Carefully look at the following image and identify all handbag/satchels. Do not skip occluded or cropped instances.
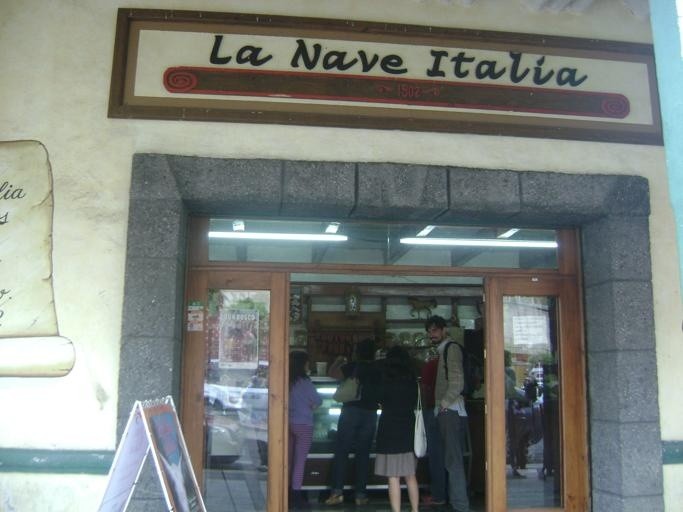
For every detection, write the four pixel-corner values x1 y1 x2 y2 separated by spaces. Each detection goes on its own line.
335 367 362 403
443 341 483 398
411 405 428 460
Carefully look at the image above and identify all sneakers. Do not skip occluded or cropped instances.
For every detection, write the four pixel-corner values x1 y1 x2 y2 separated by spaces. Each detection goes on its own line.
323 494 371 506
423 496 445 507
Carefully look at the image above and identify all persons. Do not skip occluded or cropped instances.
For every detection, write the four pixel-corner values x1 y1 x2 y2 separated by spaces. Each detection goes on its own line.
158 449 190 512
289 350 322 491
374 346 420 512
204 362 268 473
503 350 552 480
419 315 484 512
326 338 383 506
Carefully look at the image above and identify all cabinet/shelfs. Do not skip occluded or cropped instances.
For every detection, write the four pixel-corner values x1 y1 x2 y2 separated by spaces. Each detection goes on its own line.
289 294 310 352
295 384 431 490
380 297 459 362
